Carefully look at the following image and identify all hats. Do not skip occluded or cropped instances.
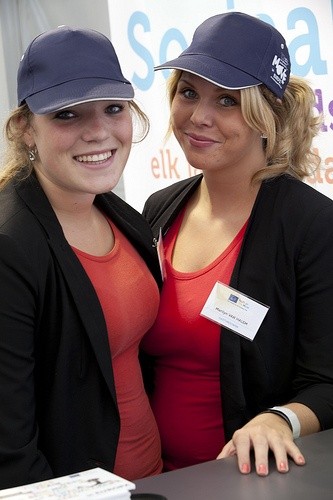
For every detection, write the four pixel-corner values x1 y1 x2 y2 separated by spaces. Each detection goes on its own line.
152 11 291 99
16 24 134 115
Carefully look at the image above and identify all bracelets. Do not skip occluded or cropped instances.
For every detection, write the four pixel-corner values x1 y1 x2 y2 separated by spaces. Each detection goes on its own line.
269 406 301 440
259 409 293 432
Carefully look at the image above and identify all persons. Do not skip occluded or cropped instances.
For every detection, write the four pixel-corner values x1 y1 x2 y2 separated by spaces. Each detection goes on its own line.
0 25 162 481
141 12 333 476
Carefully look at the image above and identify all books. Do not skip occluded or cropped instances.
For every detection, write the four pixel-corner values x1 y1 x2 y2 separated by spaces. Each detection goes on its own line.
0 467 137 500
